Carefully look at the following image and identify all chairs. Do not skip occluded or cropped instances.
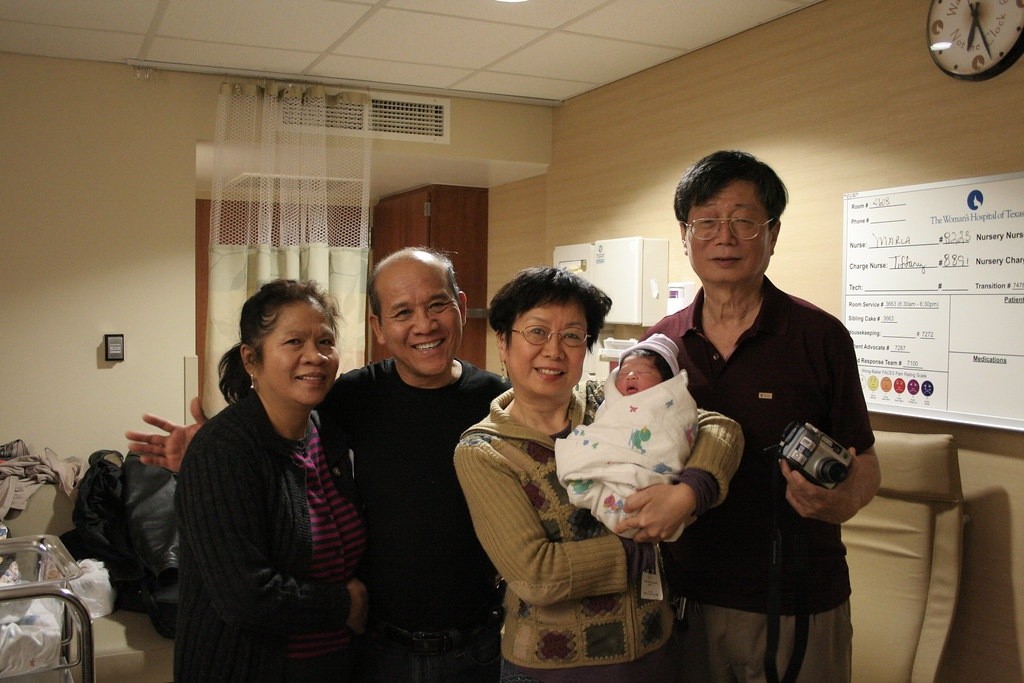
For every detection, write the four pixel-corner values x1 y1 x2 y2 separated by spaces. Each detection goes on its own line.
842 434 968 681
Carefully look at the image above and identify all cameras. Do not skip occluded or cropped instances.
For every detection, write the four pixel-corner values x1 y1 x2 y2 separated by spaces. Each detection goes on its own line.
777 420 854 490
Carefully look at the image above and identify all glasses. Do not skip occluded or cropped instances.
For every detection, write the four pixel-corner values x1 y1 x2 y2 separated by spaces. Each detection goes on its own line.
506 325 591 347
680 216 774 241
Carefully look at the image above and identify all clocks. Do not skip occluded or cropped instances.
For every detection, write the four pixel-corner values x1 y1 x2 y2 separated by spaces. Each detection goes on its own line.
928 0 1024 81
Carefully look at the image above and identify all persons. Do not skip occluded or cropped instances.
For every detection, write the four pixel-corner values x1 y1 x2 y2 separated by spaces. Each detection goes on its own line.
555 332 698 543
454 266 746 683
638 151 882 683
174 278 369 683
125 247 513 683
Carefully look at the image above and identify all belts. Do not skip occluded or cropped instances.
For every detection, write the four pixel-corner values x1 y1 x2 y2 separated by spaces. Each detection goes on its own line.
383 622 470 655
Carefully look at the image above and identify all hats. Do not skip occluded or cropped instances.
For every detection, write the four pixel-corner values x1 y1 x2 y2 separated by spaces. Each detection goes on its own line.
618 332 680 376
